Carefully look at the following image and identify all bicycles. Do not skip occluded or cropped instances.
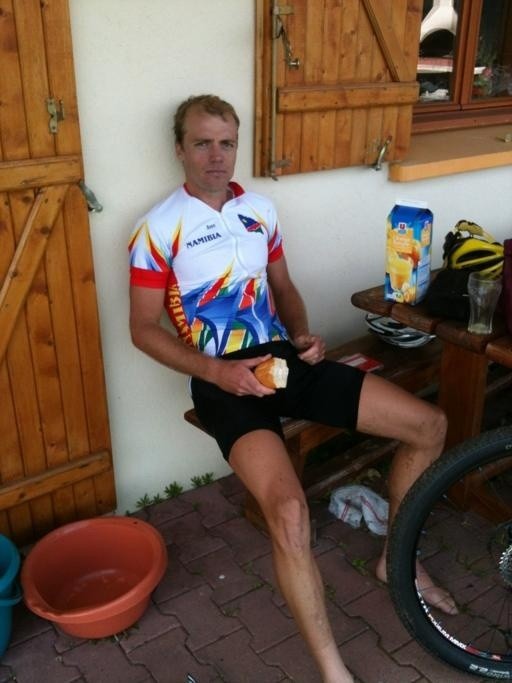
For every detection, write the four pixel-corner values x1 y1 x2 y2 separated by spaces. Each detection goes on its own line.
385 421 512 683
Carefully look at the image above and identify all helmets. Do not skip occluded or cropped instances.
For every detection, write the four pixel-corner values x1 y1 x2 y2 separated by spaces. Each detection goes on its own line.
440 220 504 289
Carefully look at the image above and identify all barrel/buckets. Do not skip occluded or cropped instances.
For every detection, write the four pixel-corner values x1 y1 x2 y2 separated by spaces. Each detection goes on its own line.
0 533 24 661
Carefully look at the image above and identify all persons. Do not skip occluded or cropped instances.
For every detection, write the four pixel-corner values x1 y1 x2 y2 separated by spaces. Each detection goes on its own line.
127 94 459 683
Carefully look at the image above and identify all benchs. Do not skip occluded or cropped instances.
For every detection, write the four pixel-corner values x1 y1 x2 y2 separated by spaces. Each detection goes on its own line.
182 336 445 549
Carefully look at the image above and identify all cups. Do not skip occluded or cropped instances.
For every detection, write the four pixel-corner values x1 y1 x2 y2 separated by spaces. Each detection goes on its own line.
466 269 503 335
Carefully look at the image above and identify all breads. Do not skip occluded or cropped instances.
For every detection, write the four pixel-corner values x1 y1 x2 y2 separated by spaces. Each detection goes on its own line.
254 357 289 389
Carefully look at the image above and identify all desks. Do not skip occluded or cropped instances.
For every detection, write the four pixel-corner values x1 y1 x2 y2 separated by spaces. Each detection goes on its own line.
350 268 512 526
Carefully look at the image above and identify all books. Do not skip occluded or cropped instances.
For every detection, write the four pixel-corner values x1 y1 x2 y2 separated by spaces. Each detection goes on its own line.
335 352 384 373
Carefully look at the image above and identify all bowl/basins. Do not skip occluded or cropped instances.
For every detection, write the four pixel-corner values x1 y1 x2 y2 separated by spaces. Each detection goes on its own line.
19 515 170 638
1 532 24 659
